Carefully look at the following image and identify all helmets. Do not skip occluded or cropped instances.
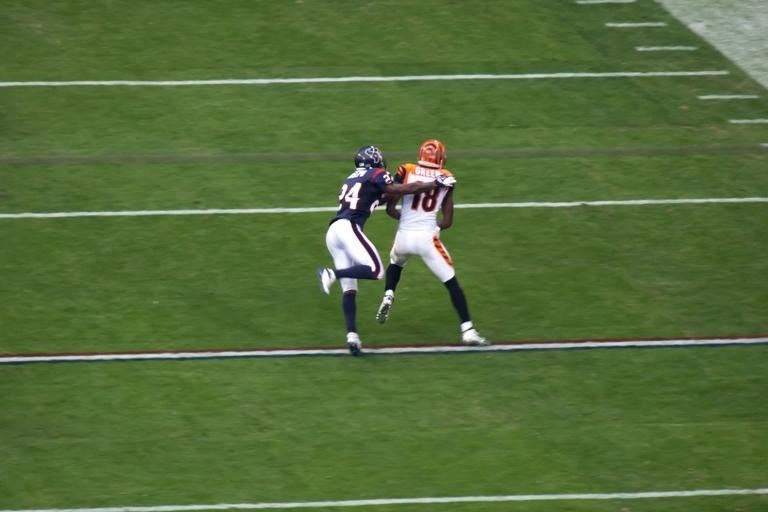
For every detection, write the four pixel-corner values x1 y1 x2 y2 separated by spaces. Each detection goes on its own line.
417 138 447 168
354 145 386 170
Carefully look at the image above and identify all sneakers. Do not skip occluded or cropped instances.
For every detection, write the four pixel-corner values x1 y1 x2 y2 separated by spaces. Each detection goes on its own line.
318 266 337 297
375 299 393 324
461 333 491 346
347 338 362 357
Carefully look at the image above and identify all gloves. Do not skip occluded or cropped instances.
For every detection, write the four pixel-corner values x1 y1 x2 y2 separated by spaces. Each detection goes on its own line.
436 174 457 188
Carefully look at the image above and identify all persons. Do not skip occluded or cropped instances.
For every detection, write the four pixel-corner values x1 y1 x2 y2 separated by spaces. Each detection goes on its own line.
375 139 490 347
319 144 459 359
